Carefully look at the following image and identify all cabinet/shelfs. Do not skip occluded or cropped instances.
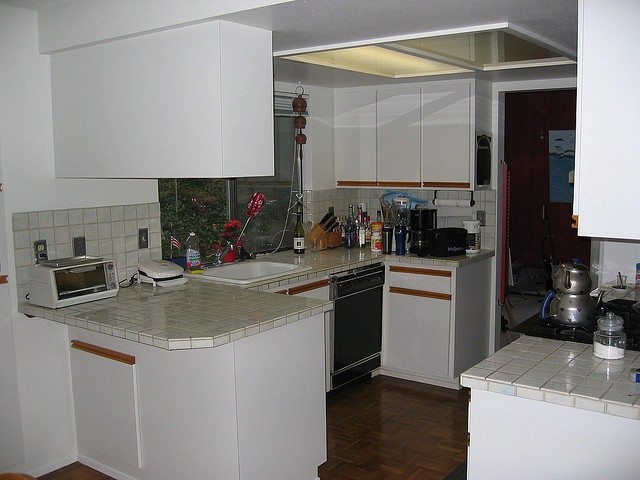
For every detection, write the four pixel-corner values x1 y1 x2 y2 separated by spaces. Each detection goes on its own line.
386 266 452 378
67 341 143 469
422 88 472 191
377 90 421 188
288 280 330 302
332 90 377 188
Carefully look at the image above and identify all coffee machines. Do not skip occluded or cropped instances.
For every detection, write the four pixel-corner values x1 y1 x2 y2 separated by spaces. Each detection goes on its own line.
408 208 437 257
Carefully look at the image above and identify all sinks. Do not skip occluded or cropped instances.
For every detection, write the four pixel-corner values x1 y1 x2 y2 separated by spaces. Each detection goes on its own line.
202 261 313 282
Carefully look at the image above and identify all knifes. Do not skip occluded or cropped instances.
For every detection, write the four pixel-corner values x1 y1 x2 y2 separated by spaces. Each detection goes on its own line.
320 212 339 234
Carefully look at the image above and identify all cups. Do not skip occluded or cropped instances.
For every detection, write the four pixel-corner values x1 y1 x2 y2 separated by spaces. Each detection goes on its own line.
617 275 627 289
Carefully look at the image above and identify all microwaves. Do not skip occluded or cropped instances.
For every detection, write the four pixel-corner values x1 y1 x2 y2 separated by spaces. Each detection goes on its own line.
28 254 121 310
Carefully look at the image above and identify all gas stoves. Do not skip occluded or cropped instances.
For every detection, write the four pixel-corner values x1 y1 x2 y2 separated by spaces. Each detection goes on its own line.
505 296 640 352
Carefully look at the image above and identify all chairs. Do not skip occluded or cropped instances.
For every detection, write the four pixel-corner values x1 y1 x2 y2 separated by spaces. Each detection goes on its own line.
506 259 541 299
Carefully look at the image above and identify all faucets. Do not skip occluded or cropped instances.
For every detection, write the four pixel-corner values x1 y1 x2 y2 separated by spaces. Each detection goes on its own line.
215 240 233 263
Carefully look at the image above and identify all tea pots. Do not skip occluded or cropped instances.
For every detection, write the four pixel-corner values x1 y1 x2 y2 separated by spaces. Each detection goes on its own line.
549 258 592 295
539 290 606 326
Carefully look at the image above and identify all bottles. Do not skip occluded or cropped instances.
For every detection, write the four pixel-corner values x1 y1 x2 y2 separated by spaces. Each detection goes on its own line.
377 210 382 222
357 205 364 223
365 215 372 244
348 204 355 224
382 226 393 254
293 202 305 254
395 225 407 256
345 219 354 248
358 222 367 248
370 232 383 253
462 220 482 254
593 311 628 359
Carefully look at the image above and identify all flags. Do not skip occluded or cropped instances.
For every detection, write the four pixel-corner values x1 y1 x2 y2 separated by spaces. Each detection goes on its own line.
171 226 182 253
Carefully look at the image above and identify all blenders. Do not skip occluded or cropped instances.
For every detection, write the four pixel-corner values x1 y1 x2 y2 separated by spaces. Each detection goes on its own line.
388 197 414 253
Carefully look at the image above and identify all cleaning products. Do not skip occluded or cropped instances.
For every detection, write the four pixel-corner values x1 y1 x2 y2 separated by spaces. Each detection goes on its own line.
186 232 202 271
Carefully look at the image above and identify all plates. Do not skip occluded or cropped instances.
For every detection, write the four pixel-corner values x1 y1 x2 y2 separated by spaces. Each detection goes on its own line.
610 285 632 293
